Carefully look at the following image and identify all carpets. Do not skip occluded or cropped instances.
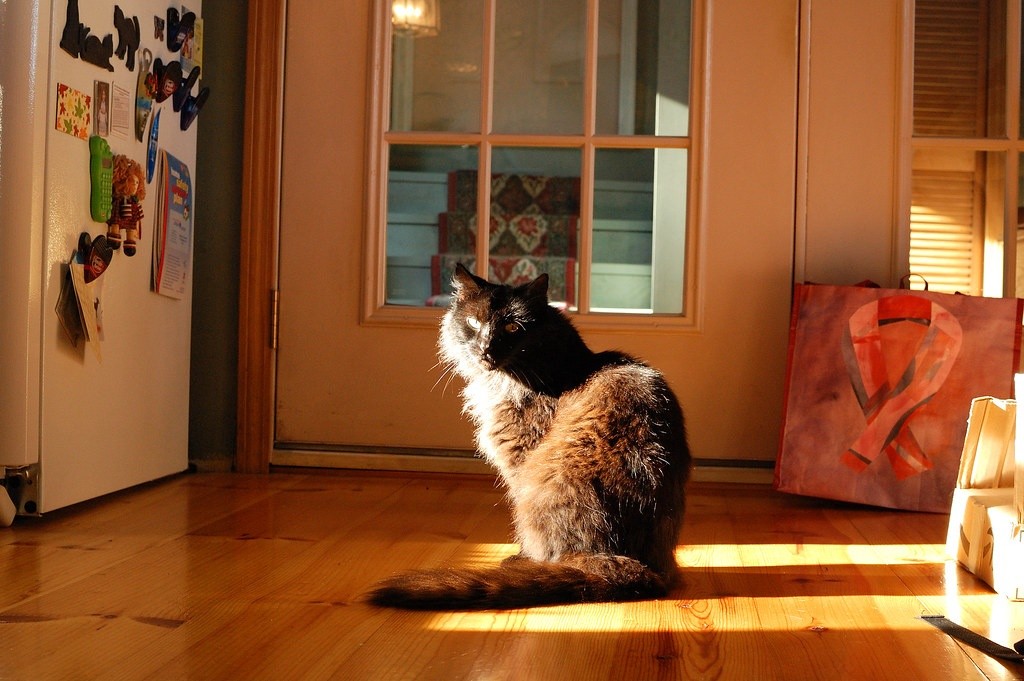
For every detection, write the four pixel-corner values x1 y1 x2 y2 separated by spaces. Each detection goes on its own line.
431 170 581 308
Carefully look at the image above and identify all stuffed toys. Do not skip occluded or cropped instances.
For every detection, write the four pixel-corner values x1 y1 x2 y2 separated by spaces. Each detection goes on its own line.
108 154 146 258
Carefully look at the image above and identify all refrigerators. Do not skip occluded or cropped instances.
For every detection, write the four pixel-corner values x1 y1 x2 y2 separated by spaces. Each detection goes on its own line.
0 1 205 521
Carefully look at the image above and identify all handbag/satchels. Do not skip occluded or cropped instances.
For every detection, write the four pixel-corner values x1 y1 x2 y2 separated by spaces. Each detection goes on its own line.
775 274 1024 512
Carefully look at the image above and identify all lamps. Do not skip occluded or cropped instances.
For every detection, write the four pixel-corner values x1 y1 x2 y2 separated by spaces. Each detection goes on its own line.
390 0 441 40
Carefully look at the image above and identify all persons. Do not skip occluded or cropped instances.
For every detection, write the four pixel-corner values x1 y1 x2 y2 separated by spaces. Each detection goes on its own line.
182 28 194 59
98 86 108 135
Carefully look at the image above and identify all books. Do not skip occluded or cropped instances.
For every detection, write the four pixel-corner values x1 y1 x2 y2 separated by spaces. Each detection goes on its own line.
153 149 192 299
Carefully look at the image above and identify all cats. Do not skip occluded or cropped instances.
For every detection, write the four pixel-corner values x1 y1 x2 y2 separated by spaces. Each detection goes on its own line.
355 263 691 612
